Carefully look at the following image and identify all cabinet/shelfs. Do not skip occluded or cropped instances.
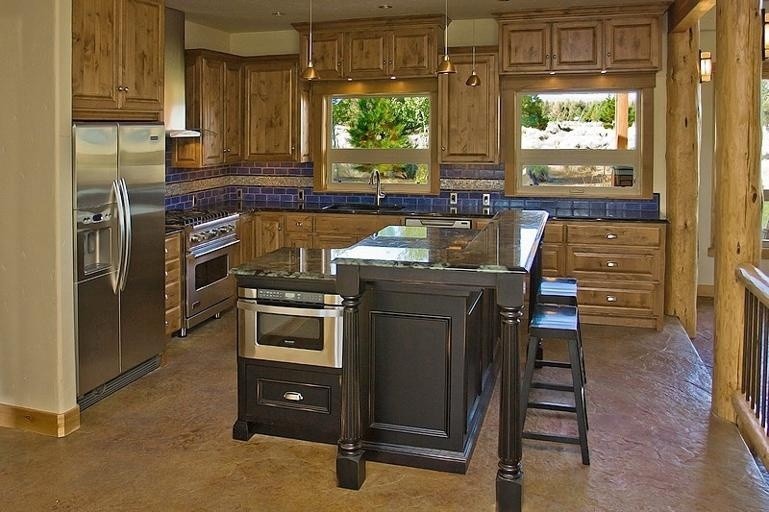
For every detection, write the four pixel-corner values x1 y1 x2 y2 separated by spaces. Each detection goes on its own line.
603 15 663 73
498 18 603 73
245 57 310 164
255 209 284 257
559 221 669 331
164 234 184 334
436 53 498 164
315 212 404 250
285 211 313 250
171 48 243 169
344 24 444 81
240 210 256 264
72 0 166 123
540 223 568 277
300 32 344 80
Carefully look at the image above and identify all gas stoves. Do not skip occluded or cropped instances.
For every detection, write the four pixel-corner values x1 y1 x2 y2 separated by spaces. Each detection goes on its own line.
165 207 234 227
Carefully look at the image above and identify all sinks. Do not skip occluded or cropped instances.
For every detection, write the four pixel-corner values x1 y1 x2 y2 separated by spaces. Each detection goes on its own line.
379 206 404 213
322 204 380 212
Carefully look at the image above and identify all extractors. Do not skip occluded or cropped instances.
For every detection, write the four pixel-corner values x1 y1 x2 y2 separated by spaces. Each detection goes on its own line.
165 20 202 139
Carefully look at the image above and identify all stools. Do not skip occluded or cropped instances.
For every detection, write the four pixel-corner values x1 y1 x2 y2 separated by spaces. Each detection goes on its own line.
521 305 590 464
536 277 587 385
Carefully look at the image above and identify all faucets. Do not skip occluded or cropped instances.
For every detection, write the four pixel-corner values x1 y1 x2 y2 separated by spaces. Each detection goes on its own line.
370 169 385 206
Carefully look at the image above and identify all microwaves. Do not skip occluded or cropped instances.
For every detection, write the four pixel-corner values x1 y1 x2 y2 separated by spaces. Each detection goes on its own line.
236 290 344 373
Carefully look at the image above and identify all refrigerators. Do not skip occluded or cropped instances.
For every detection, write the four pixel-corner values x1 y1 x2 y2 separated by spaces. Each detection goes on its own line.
71 120 166 413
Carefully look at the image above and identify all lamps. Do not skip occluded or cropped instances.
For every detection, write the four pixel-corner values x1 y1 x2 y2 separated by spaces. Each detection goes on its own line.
464 18 481 86
700 51 712 81
301 0 321 80
435 1 458 75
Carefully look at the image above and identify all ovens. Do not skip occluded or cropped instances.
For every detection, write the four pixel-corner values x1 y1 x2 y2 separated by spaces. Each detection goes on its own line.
183 217 240 330
